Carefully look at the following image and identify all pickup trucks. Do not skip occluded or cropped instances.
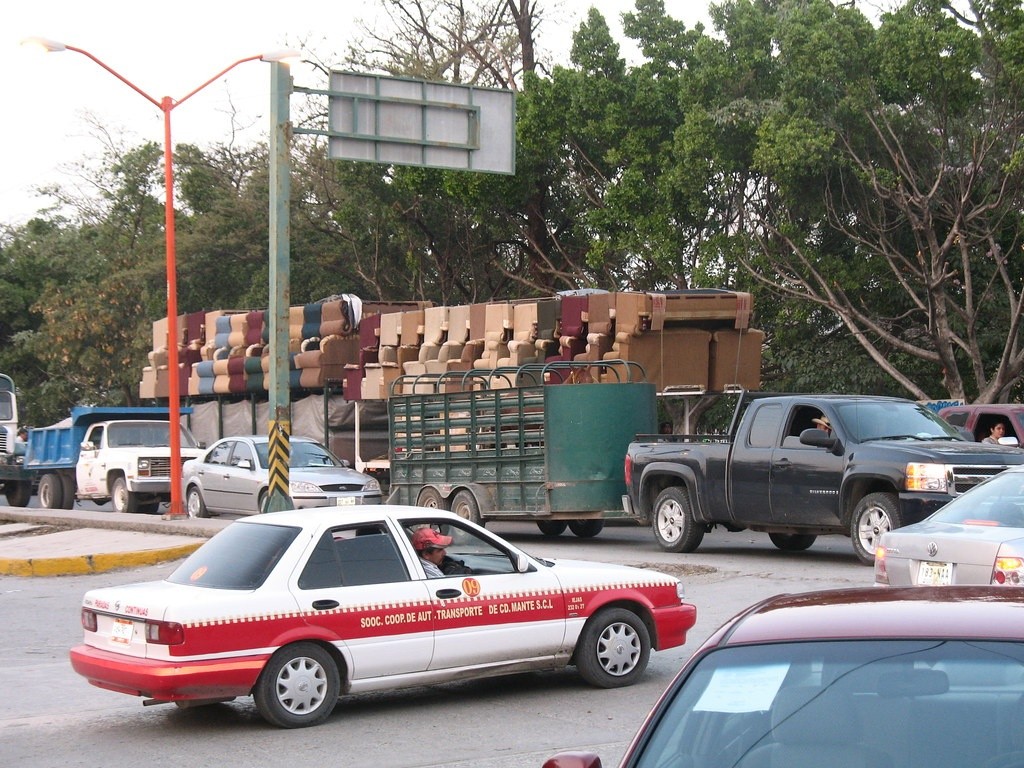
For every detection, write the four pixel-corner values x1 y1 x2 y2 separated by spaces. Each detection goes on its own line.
621 392 1024 568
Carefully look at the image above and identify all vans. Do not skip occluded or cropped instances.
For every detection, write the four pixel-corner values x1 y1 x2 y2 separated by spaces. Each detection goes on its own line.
935 403 1024 452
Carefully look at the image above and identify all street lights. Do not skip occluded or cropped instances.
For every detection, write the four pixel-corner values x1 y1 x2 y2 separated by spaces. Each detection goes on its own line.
17 36 308 519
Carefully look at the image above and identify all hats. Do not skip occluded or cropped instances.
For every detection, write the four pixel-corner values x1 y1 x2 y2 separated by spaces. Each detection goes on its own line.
412 528 452 549
812 415 832 430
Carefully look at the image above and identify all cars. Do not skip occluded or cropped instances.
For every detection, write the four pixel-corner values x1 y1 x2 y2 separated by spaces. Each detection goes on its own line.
69 504 700 729
542 586 1024 768
180 435 382 517
870 460 1024 590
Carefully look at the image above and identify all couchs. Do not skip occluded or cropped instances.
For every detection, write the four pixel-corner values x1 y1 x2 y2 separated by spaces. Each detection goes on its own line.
139 287 764 403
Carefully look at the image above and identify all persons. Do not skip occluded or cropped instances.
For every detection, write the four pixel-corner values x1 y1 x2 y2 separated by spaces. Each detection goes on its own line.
982 419 1006 445
817 416 832 437
356 526 452 579
659 422 673 434
17 428 28 442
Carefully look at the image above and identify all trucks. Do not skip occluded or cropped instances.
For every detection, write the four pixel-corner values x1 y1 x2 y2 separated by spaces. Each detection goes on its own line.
21 406 213 514
0 373 34 509
